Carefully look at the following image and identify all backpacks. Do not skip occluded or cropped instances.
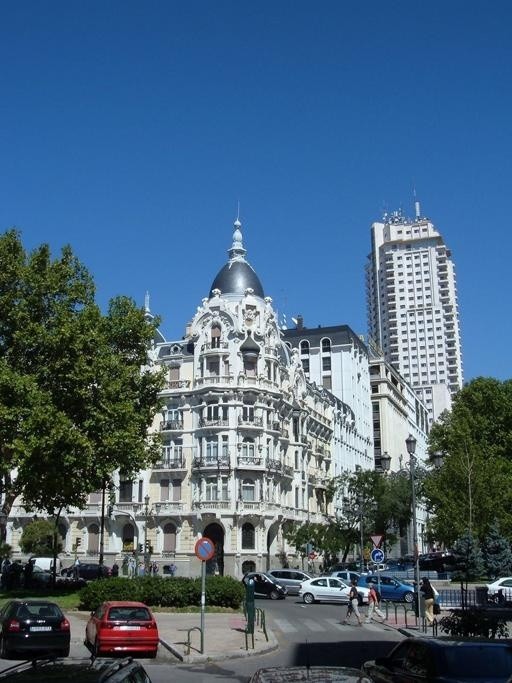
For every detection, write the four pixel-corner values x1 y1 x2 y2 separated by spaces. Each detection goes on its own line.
373 588 381 603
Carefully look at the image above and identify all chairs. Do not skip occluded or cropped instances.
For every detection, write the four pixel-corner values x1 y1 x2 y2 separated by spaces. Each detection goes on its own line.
131 611 146 618
38 608 51 617
110 611 120 618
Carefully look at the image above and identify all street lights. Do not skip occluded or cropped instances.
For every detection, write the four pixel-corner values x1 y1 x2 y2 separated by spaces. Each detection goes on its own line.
380 434 444 631
129 495 161 576
342 492 381 573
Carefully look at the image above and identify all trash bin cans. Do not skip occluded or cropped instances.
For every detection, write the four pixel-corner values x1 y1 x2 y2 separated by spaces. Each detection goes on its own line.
475 586 488 605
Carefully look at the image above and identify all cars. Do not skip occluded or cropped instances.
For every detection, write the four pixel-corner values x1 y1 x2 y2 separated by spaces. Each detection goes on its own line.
62 562 111 580
486 576 512 604
242 563 425 604
0 598 158 683
20 565 53 584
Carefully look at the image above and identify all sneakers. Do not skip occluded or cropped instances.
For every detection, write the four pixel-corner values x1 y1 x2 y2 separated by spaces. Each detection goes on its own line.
342 616 387 625
421 620 437 627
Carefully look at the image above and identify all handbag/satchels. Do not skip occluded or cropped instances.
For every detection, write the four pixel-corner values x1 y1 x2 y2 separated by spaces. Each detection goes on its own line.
434 603 441 614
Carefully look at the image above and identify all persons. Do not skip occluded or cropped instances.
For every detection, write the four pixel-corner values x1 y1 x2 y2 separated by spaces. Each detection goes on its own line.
365 581 385 624
340 579 363 625
24 557 36 588
72 554 82 578
419 576 436 627
111 558 179 579
